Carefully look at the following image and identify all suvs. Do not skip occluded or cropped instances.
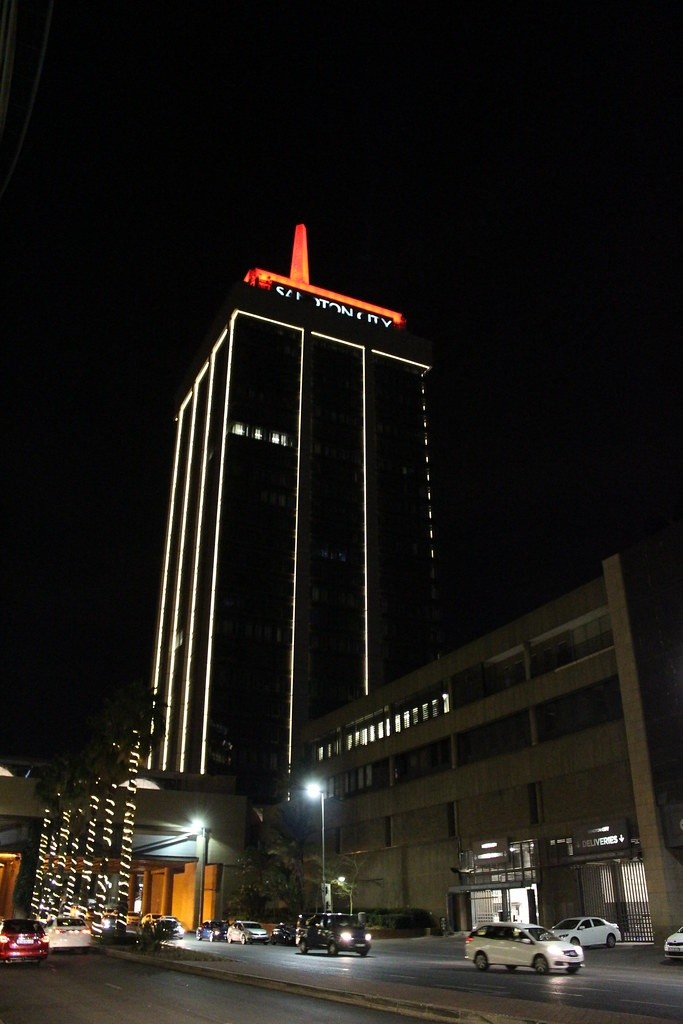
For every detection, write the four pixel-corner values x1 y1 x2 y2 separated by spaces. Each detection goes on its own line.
295 912 372 957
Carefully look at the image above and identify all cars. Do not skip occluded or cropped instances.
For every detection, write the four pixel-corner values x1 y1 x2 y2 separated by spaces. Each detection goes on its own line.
0 918 51 966
465 922 586 976
270 924 297 947
197 920 230 943
539 917 622 951
40 916 92 955
226 921 269 945
664 928 683 960
142 913 185 940
102 908 140 926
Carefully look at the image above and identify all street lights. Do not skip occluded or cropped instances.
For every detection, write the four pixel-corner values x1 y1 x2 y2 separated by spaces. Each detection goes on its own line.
306 782 328 915
190 817 208 928
